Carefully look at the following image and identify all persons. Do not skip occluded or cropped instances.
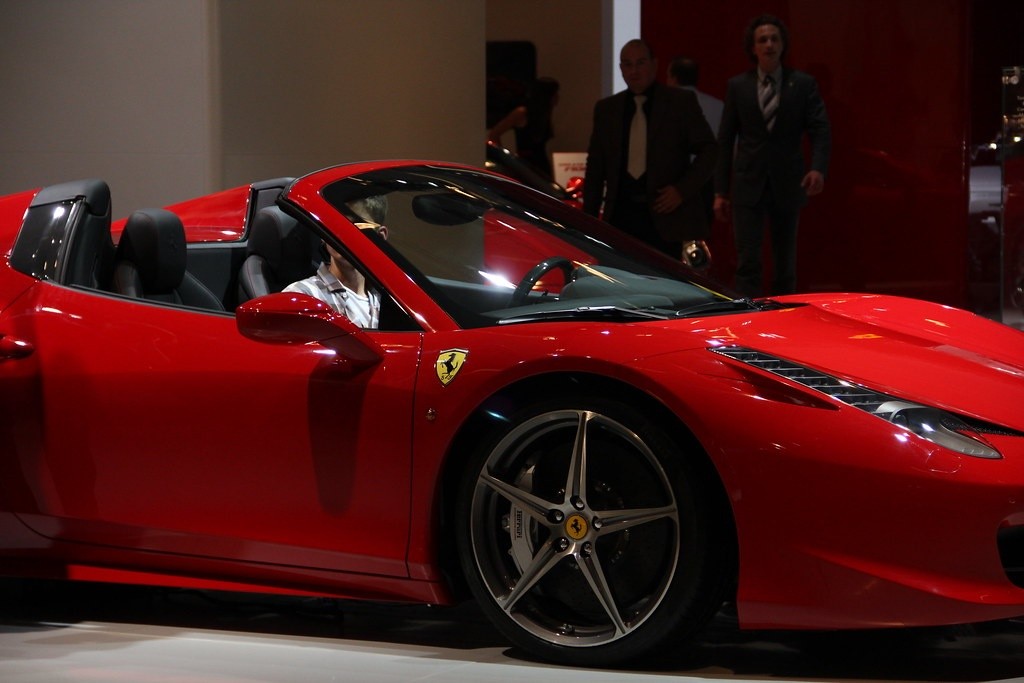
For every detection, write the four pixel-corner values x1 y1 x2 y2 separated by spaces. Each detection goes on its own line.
663 57 726 263
581 38 717 263
280 194 389 328
487 76 560 185
711 16 833 299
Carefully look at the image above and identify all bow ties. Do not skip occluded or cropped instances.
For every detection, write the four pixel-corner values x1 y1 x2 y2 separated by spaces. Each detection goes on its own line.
626 95 647 180
762 74 779 133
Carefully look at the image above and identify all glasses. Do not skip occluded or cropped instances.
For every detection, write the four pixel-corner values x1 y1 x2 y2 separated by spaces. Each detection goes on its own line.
619 57 649 70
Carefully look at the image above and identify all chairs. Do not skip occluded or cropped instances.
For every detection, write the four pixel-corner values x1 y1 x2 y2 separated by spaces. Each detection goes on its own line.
235 205 325 308
108 207 226 317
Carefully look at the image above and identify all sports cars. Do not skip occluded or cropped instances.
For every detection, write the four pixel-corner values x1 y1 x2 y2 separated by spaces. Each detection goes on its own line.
2 158 1024 663
